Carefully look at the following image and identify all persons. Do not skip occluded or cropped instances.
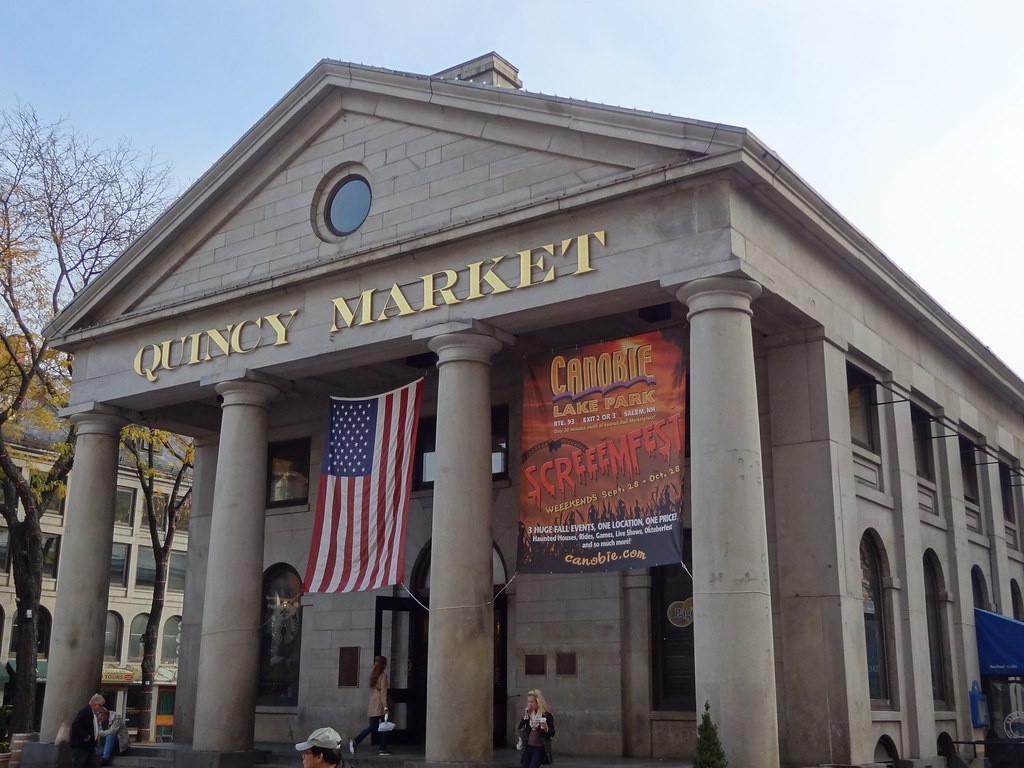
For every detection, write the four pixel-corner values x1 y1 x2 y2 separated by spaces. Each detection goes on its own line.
349 656 392 756
69 693 105 768
294 726 355 768
517 688 556 768
94 707 130 768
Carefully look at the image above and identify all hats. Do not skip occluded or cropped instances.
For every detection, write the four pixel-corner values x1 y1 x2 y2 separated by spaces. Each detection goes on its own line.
295 727 342 751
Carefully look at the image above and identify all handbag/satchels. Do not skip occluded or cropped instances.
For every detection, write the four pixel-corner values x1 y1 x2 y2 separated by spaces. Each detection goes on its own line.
515 737 523 751
378 718 396 731
54 720 72 745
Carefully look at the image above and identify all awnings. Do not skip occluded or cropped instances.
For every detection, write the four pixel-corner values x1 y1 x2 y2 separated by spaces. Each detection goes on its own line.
974 606 1024 678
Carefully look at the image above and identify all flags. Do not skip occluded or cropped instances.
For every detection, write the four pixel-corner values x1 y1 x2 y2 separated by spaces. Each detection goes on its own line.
300 377 423 593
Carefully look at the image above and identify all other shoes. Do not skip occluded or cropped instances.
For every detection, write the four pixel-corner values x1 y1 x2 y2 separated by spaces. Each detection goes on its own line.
378 750 392 756
348 740 356 755
